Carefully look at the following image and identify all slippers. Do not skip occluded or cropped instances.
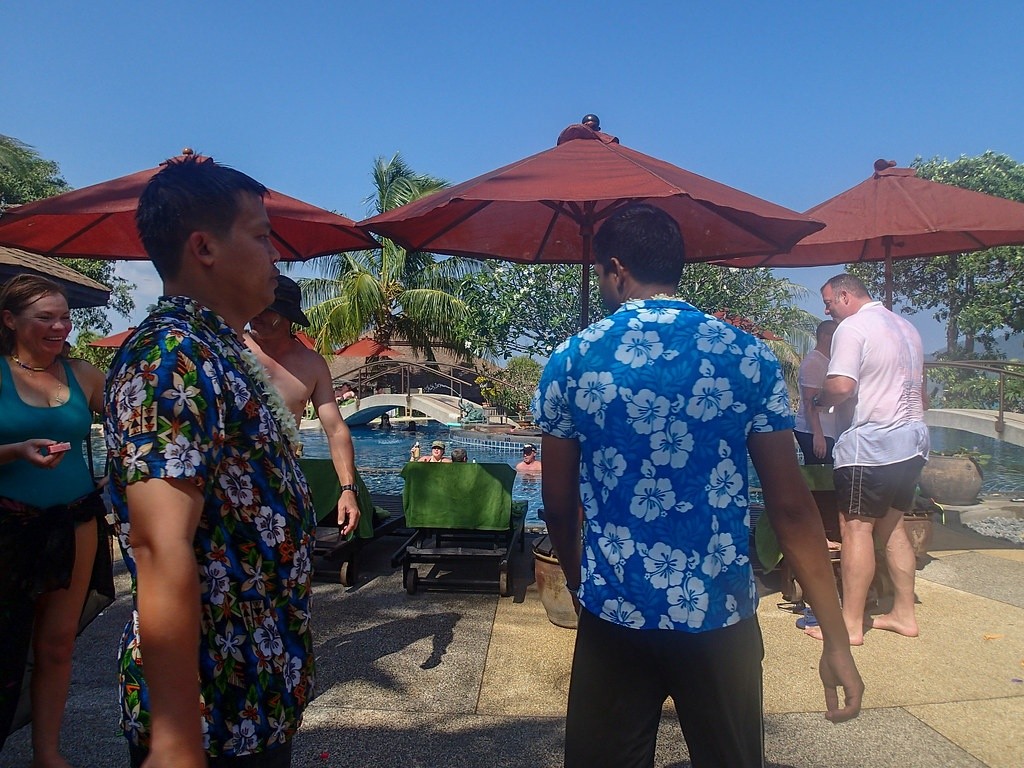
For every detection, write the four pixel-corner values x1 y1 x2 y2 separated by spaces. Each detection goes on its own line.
794 605 820 629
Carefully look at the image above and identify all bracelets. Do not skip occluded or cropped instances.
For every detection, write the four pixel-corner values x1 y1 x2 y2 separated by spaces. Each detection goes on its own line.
341 482 359 497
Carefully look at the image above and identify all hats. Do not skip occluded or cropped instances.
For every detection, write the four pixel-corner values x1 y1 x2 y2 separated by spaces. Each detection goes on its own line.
520 444 537 452
262 275 309 326
430 441 445 449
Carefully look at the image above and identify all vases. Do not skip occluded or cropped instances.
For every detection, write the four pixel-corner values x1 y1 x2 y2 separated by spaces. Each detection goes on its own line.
900 515 932 570
533 549 579 629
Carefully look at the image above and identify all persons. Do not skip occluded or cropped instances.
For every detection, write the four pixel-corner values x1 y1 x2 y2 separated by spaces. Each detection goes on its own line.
516 443 541 471
411 440 468 462
103 150 317 768
792 320 839 465
802 274 931 648
242 274 361 535
0 274 108 768
533 202 866 768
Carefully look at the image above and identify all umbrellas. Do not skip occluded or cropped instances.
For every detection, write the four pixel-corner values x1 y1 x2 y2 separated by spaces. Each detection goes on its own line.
712 310 784 339
708 158 1024 312
334 337 407 356
353 113 829 332
0 147 387 263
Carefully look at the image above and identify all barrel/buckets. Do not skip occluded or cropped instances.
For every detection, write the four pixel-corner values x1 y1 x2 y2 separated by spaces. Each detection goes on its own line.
532 547 579 630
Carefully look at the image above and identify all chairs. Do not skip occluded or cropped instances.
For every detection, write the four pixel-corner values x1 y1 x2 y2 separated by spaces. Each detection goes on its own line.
295 458 411 587
747 464 878 614
391 462 529 596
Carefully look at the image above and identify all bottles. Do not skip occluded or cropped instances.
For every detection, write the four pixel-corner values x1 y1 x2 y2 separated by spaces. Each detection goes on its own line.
413 441 420 458
473 457 476 463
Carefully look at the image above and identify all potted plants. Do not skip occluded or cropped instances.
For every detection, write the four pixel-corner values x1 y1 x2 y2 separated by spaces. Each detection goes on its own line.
918 444 992 506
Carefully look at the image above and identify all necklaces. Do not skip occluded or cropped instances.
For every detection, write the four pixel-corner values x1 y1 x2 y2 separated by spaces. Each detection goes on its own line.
19 363 66 403
11 352 58 371
